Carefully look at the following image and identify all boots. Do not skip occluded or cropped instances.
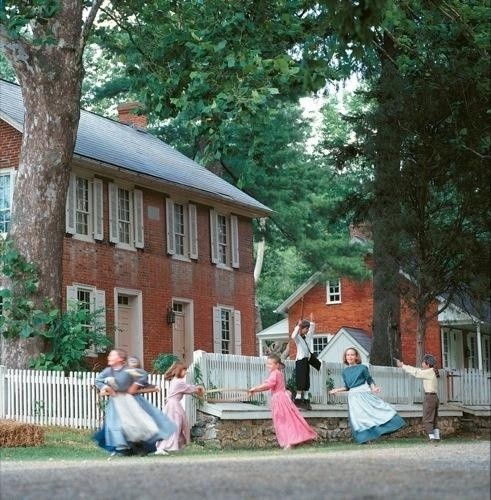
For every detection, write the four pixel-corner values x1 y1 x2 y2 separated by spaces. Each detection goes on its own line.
294 399 312 410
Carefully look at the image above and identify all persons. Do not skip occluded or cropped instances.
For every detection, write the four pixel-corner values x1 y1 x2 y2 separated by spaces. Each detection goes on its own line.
91 355 162 457
328 346 408 446
391 352 444 442
92 347 180 459
248 353 322 451
152 359 207 457
290 318 318 411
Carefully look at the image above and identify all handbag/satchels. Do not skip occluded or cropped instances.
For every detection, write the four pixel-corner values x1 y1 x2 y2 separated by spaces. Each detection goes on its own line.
308 353 321 370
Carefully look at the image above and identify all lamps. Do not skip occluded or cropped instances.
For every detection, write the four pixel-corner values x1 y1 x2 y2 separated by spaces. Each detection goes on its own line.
166 301 176 325
